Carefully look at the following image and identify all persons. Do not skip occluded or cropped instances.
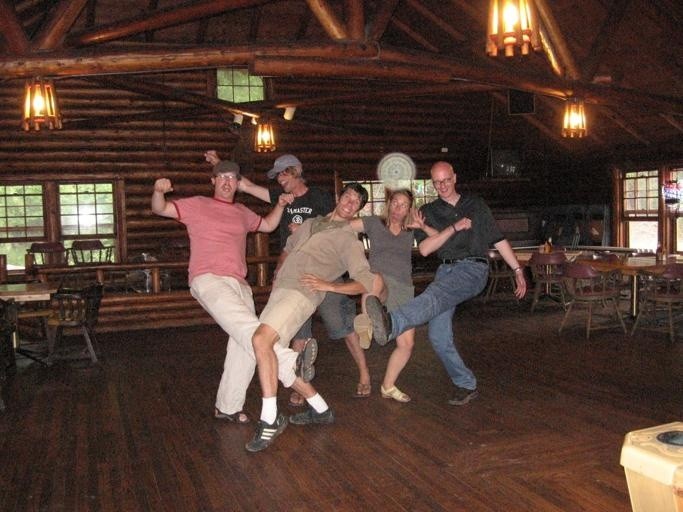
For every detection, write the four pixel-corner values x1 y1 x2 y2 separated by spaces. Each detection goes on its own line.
204 148 373 406
243 183 373 453
151 161 319 424
365 161 528 406
289 189 438 403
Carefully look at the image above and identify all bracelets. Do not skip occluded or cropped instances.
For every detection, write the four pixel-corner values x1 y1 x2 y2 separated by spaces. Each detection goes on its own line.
449 223 456 234
514 267 523 273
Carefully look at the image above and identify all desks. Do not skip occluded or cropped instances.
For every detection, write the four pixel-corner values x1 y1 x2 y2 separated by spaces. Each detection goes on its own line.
510 251 583 300
0 282 62 352
573 253 683 318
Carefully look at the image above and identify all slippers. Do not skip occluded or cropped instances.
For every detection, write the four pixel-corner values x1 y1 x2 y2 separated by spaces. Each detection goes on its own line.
287 390 308 409
350 382 372 399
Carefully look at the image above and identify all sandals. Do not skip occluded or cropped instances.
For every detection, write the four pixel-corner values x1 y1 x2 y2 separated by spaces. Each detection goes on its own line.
380 383 411 403
213 408 251 424
295 338 319 383
353 313 372 350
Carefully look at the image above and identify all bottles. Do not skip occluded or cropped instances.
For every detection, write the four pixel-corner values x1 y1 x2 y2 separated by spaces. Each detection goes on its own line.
548 238 552 253
544 235 550 253
656 241 662 261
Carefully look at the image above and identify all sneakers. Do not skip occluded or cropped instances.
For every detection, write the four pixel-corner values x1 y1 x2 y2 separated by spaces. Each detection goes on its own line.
448 387 480 406
244 411 290 453
289 406 336 425
365 294 392 348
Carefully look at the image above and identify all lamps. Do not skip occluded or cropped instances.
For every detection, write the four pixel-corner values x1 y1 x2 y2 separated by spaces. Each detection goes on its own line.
485 0 541 61
253 118 275 154
562 94 588 140
21 76 63 133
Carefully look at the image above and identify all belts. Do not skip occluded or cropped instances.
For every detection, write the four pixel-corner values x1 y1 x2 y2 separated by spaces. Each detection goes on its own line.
445 257 488 264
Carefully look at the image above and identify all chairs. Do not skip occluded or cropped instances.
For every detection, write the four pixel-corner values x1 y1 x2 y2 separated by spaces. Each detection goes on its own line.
537 245 566 254
604 254 629 314
630 263 683 342
482 250 524 307
558 263 627 340
635 252 656 257
27 242 69 283
71 241 116 264
530 251 570 316
47 282 105 363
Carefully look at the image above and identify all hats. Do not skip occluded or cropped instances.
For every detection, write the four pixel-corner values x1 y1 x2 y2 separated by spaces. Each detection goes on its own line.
267 153 302 180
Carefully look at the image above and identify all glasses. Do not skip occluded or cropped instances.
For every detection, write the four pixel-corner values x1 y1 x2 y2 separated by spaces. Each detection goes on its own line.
215 174 238 181
433 176 454 186
